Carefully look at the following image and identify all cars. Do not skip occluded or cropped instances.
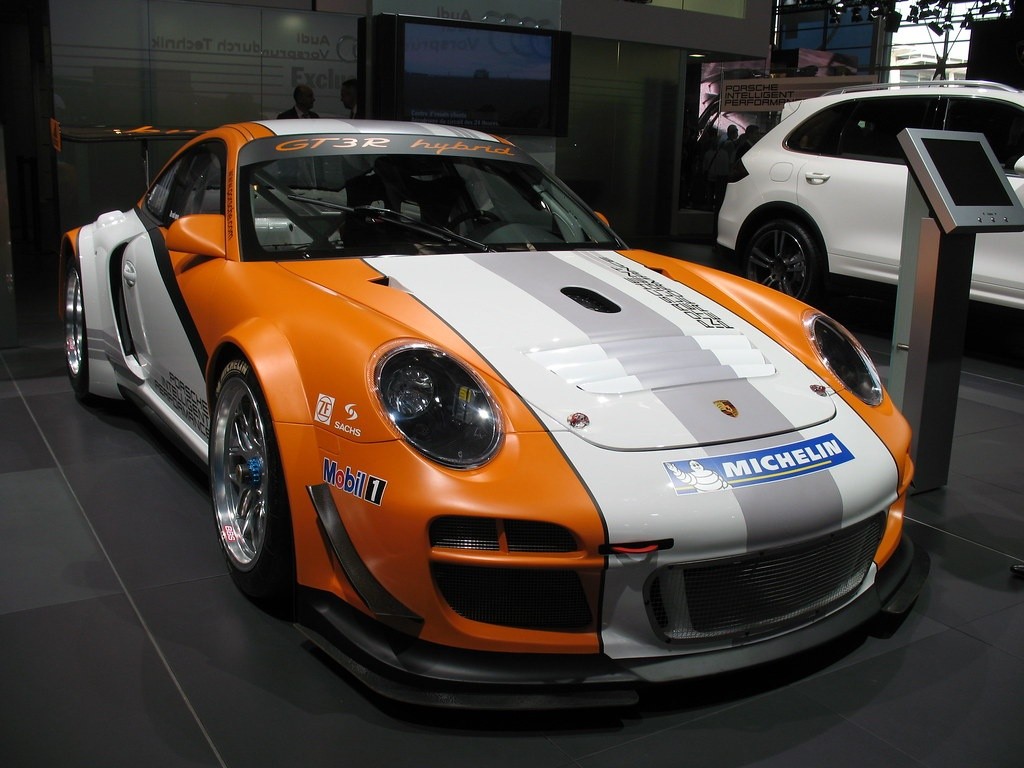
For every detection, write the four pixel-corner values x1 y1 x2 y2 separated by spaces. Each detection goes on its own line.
57 118 929 714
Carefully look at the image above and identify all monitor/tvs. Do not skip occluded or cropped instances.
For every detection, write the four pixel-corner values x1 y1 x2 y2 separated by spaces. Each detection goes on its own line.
896 128 1024 235
372 13 572 138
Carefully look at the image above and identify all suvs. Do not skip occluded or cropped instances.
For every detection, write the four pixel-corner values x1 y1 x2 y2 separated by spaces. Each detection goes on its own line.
711 79 1024 310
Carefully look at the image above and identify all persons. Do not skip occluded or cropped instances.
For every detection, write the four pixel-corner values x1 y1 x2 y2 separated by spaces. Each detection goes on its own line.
277 84 319 118
702 124 762 211
341 79 358 116
